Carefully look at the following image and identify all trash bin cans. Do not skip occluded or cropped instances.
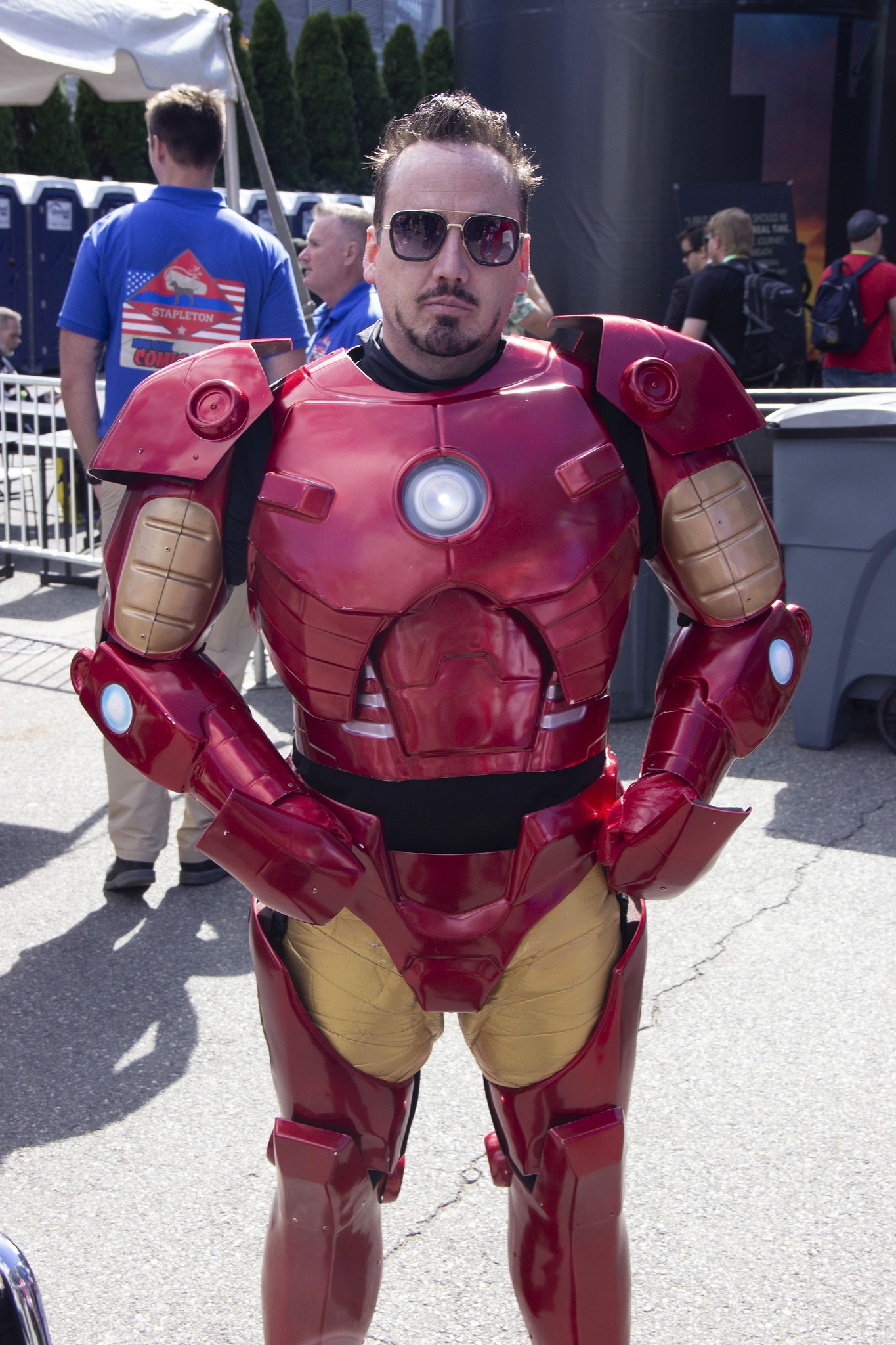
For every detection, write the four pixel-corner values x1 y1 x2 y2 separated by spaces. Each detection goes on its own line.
608 558 671 723
766 395 896 751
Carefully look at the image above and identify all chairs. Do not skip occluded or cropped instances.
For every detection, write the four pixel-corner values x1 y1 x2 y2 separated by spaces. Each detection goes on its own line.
0 468 38 546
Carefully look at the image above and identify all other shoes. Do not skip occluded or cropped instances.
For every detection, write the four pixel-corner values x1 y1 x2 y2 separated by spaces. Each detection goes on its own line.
104 857 157 889
179 858 230 885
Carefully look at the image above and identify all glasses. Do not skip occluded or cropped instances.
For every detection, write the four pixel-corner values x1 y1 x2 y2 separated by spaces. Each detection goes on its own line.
381 210 523 267
681 248 697 259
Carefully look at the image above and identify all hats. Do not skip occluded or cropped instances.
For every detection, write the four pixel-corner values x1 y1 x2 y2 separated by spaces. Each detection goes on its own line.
847 210 888 242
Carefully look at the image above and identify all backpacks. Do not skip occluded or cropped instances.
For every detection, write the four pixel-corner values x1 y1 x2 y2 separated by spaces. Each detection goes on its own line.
706 259 805 388
810 253 890 354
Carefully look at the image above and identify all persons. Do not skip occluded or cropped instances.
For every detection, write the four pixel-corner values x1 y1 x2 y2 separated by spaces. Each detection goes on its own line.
58 84 814 1345
0 307 101 523
660 209 896 387
